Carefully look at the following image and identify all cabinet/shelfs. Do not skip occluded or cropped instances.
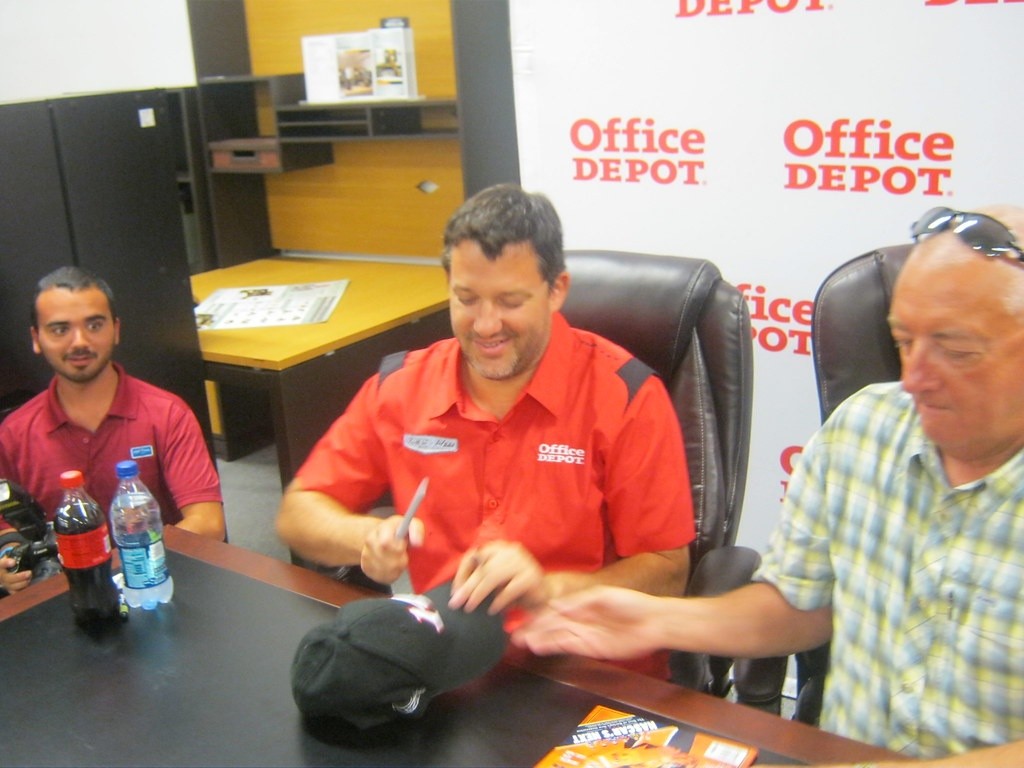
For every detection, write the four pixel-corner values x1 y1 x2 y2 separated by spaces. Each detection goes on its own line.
183 0 520 564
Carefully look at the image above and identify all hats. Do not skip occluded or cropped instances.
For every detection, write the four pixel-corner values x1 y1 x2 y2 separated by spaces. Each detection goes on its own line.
290 579 506 734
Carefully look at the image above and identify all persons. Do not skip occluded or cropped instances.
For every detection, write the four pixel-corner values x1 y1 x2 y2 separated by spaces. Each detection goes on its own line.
513 203 1024 768
275 183 698 682
0 265 226 596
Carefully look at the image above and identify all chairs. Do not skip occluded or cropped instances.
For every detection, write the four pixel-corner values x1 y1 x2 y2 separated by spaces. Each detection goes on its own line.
736 238 912 736
559 250 763 695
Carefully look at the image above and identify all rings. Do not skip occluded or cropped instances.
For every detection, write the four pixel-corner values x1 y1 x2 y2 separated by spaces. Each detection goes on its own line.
462 552 480 571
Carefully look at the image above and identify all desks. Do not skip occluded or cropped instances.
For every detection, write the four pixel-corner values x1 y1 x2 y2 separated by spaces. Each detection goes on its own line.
0 521 912 768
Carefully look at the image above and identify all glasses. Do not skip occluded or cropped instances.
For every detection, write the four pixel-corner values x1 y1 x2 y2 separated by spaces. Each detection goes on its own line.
911 206 1024 261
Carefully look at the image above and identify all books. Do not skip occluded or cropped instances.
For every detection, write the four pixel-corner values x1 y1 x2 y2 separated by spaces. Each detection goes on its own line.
532 705 758 768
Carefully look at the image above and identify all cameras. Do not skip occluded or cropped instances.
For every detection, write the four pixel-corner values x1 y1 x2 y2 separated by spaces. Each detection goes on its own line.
0 479 64 594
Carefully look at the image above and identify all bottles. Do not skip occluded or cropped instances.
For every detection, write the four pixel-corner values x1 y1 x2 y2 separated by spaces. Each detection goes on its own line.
109 459 173 610
53 470 120 639
